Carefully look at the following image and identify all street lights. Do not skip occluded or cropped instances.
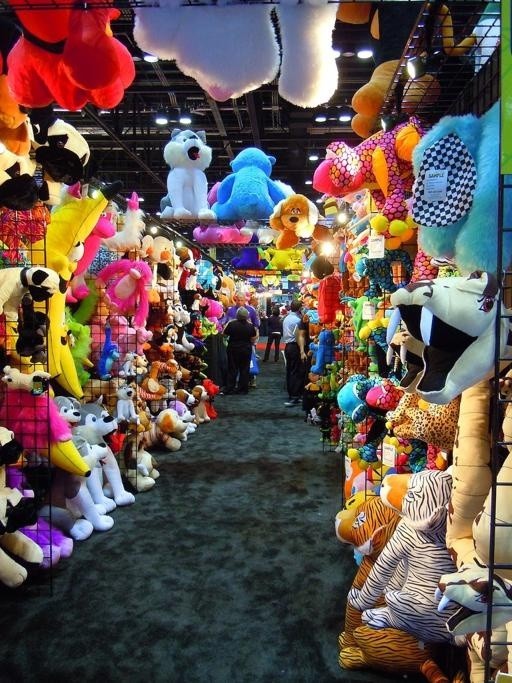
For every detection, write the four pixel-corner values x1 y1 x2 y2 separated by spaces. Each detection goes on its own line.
249 349 259 375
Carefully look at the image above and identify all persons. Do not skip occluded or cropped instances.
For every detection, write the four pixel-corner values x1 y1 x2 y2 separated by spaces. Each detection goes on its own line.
281 300 302 406
262 307 283 362
220 293 259 388
296 307 323 422
221 306 259 396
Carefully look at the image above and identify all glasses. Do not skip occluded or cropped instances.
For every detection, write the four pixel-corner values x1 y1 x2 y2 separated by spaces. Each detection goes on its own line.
379 113 411 130
407 52 444 78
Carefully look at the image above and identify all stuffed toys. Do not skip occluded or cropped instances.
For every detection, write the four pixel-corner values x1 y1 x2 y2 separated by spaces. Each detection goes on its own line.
212 147 287 223
2 74 258 587
131 3 340 108
271 56 509 683
6 1 135 114
336 2 479 59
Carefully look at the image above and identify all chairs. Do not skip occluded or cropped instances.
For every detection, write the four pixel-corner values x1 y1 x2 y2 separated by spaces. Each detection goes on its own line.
283 398 302 406
